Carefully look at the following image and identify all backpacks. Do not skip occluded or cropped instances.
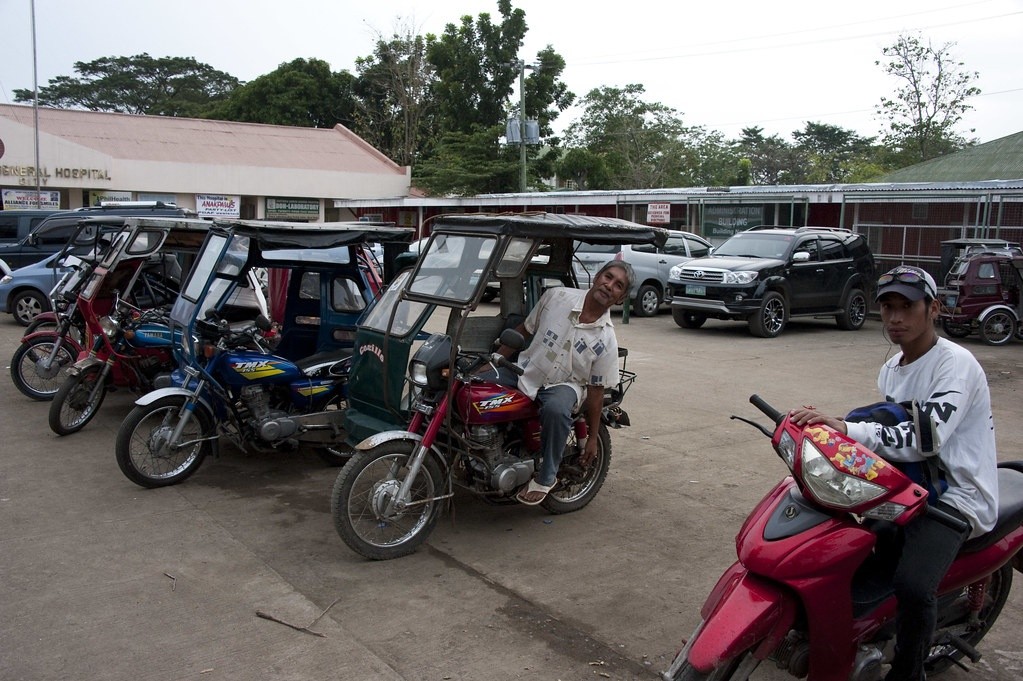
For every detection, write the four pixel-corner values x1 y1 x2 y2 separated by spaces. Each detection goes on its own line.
842 395 950 505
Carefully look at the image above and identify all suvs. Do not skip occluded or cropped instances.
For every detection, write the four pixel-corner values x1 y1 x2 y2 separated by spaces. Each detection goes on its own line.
665 224 879 338
0 201 198 272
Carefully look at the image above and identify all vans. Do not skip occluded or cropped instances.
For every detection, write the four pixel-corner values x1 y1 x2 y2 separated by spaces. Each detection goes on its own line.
0 211 68 243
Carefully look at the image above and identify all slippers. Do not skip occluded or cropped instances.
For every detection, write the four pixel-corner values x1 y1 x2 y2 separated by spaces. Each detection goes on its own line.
516 476 558 505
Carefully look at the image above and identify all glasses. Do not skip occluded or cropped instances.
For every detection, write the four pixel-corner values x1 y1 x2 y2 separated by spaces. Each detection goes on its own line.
877 273 937 299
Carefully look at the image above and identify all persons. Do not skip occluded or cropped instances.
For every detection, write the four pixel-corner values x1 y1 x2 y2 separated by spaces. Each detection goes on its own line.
790 265 999 681
475 260 636 505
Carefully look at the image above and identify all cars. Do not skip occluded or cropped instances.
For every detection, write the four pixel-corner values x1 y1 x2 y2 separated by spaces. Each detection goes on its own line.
0 247 89 328
572 232 716 316
939 252 1023 346
373 237 431 281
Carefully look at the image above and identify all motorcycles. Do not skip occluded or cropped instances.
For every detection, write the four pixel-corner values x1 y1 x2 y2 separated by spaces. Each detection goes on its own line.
10 213 673 561
658 394 1023 681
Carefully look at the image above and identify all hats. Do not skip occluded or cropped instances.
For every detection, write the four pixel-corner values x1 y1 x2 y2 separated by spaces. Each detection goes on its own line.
875 265 938 301
